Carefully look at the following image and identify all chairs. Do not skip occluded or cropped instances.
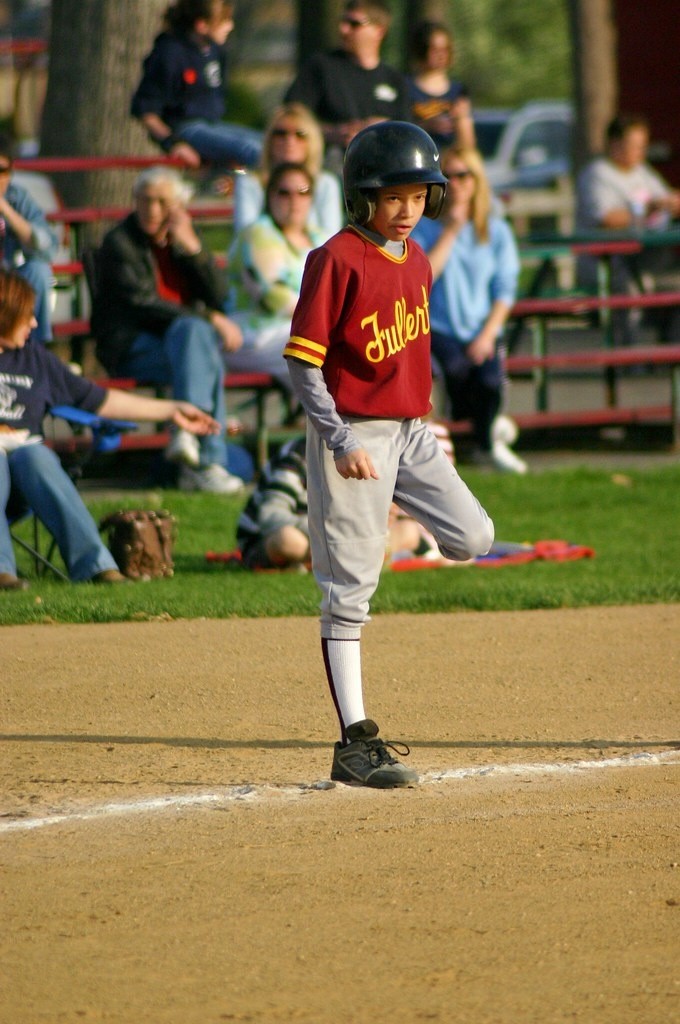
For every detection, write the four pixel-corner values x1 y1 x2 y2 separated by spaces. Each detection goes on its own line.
10 406 139 584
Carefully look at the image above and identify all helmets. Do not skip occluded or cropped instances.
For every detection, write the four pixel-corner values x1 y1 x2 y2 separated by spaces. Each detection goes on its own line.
343 121 450 227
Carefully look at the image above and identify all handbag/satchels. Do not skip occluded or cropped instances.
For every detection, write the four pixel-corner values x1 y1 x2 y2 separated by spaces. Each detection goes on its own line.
98 508 176 582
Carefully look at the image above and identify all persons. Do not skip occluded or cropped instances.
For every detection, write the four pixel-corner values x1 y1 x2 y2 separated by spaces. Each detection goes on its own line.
128 0 267 192
235 421 455 569
572 114 680 340
217 101 345 426
0 272 222 591
93 165 245 495
0 0 65 348
282 0 404 174
400 20 476 155
409 144 528 477
281 120 496 789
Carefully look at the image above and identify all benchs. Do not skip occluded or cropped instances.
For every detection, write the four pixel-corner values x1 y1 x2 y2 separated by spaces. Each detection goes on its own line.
11 158 680 468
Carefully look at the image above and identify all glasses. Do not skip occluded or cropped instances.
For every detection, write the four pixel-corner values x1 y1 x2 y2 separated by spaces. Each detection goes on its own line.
443 171 469 179
342 16 371 28
274 188 312 197
272 129 306 137
0 167 9 173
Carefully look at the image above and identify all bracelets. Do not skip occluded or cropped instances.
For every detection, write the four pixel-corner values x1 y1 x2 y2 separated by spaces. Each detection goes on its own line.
159 132 183 151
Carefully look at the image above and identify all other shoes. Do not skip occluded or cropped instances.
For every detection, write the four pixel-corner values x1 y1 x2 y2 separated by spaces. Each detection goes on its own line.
489 442 526 474
490 414 518 444
94 570 125 582
0 573 27 590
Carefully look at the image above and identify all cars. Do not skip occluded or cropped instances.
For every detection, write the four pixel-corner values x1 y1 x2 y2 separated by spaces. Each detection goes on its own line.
468 99 576 197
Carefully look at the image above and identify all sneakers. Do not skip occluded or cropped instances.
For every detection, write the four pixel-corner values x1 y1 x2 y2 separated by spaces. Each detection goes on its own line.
331 719 419 789
178 463 243 493
166 429 201 467
